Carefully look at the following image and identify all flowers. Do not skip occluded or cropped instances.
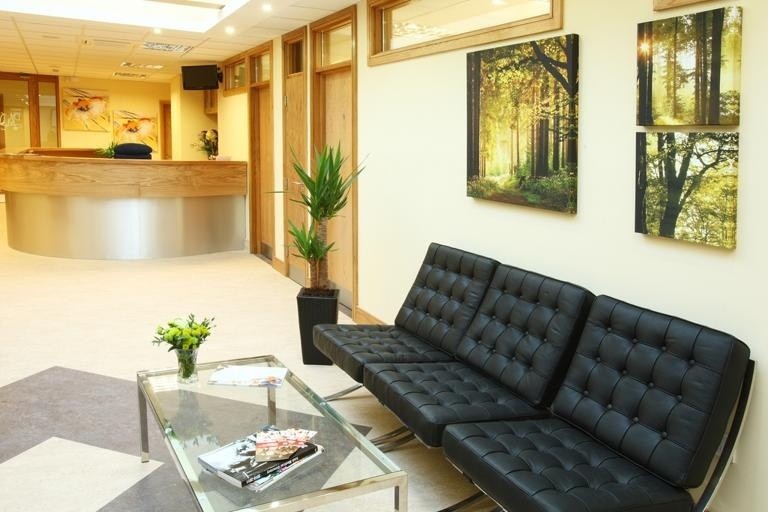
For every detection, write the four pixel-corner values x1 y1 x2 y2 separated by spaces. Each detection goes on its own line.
192 130 219 159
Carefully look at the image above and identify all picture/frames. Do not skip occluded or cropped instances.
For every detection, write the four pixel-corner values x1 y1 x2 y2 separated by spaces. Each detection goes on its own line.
634 5 741 126
635 131 739 251
112 107 159 153
63 86 111 131
466 34 579 215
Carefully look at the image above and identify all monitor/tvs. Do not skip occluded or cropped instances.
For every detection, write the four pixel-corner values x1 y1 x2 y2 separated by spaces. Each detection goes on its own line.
181 64 223 90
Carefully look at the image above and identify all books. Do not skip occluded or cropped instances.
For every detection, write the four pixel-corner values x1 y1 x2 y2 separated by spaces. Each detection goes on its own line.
198 424 324 493
209 364 288 388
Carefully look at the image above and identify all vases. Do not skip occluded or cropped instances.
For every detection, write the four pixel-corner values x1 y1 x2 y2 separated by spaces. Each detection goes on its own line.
98 142 117 158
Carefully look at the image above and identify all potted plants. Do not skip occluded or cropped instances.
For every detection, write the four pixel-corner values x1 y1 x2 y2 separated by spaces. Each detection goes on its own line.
265 140 367 364
156 313 216 382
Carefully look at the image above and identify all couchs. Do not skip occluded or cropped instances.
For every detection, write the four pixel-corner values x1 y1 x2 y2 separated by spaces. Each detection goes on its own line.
311 243 499 401
362 262 595 452
437 296 755 512
